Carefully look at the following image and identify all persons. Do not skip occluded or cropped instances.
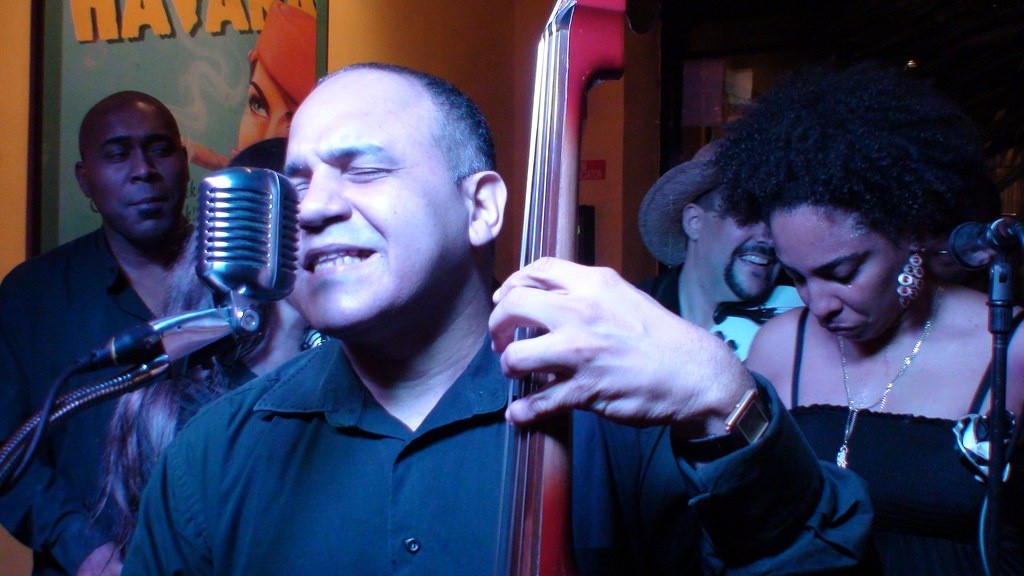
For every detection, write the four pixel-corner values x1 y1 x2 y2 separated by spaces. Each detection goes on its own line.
0 91 191 576
612 134 809 363
118 62 880 576
705 55 1024 576
96 137 312 545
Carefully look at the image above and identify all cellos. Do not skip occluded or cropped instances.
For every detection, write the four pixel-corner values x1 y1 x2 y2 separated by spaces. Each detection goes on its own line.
494 0 624 576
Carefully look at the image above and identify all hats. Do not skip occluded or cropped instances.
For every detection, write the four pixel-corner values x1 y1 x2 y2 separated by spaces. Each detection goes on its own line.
247 0 317 104
638 137 730 267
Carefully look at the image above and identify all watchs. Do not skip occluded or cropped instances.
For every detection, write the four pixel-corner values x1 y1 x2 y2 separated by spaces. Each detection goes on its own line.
680 374 770 464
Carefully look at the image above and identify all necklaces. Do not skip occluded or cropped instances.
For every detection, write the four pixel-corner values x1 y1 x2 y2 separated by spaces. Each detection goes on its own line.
837 278 946 469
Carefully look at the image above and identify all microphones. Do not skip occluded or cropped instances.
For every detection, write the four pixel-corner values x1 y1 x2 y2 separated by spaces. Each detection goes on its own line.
948 216 1020 270
195 166 301 304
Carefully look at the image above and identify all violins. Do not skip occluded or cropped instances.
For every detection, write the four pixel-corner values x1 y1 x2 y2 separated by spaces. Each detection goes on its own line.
707 284 809 363
